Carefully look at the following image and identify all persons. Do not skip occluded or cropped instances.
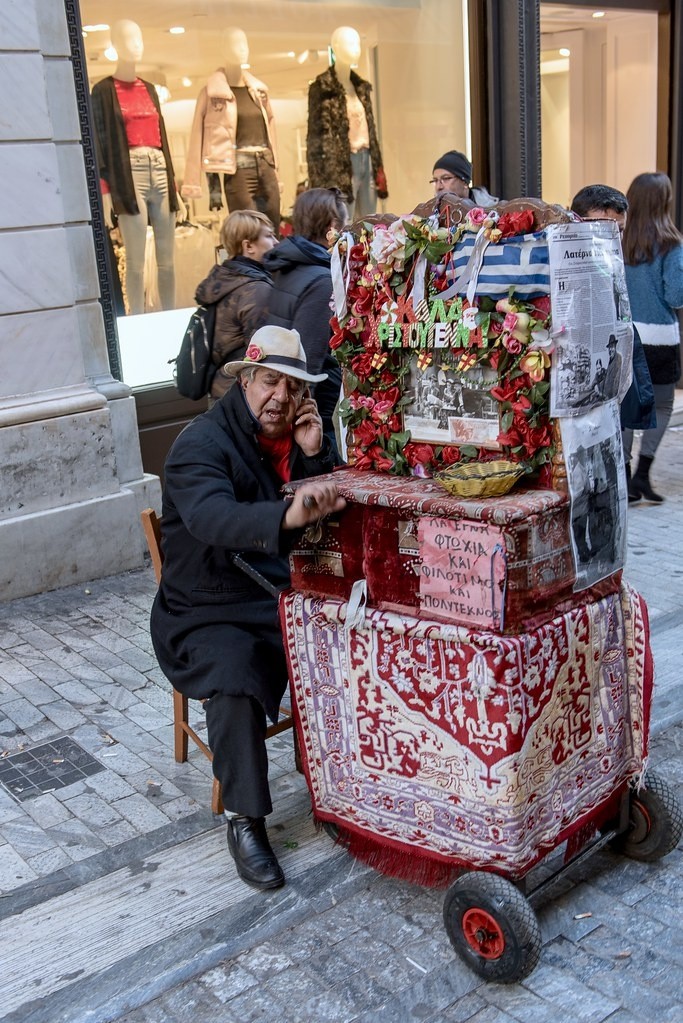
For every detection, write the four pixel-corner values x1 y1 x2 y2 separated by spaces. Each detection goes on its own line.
195 210 280 410
432 149 499 208
620 173 683 503
613 272 623 321
588 335 622 402
91 19 187 316
149 325 347 890
182 27 283 225
259 188 349 465
570 184 658 431
306 25 389 226
570 439 619 564
415 364 487 430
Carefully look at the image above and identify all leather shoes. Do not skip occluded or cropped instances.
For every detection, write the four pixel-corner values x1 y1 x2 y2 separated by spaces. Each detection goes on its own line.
227 816 285 890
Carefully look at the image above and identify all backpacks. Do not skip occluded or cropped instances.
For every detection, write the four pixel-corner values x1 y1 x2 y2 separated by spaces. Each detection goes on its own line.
168 302 223 400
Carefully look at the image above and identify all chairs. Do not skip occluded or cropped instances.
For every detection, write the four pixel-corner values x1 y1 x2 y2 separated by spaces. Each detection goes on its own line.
142 507 304 812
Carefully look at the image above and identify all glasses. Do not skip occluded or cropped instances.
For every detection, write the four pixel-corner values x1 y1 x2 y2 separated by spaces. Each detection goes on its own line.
428 176 457 185
327 188 341 195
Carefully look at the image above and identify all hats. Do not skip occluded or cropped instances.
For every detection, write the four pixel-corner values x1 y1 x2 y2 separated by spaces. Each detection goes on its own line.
606 334 619 348
225 325 329 383
432 150 473 184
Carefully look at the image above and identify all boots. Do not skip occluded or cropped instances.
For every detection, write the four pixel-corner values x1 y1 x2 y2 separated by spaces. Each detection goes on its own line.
624 461 642 502
631 455 663 504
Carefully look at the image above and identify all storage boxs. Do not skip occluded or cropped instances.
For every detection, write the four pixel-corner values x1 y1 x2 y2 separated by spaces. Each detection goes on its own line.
281 468 627 635
